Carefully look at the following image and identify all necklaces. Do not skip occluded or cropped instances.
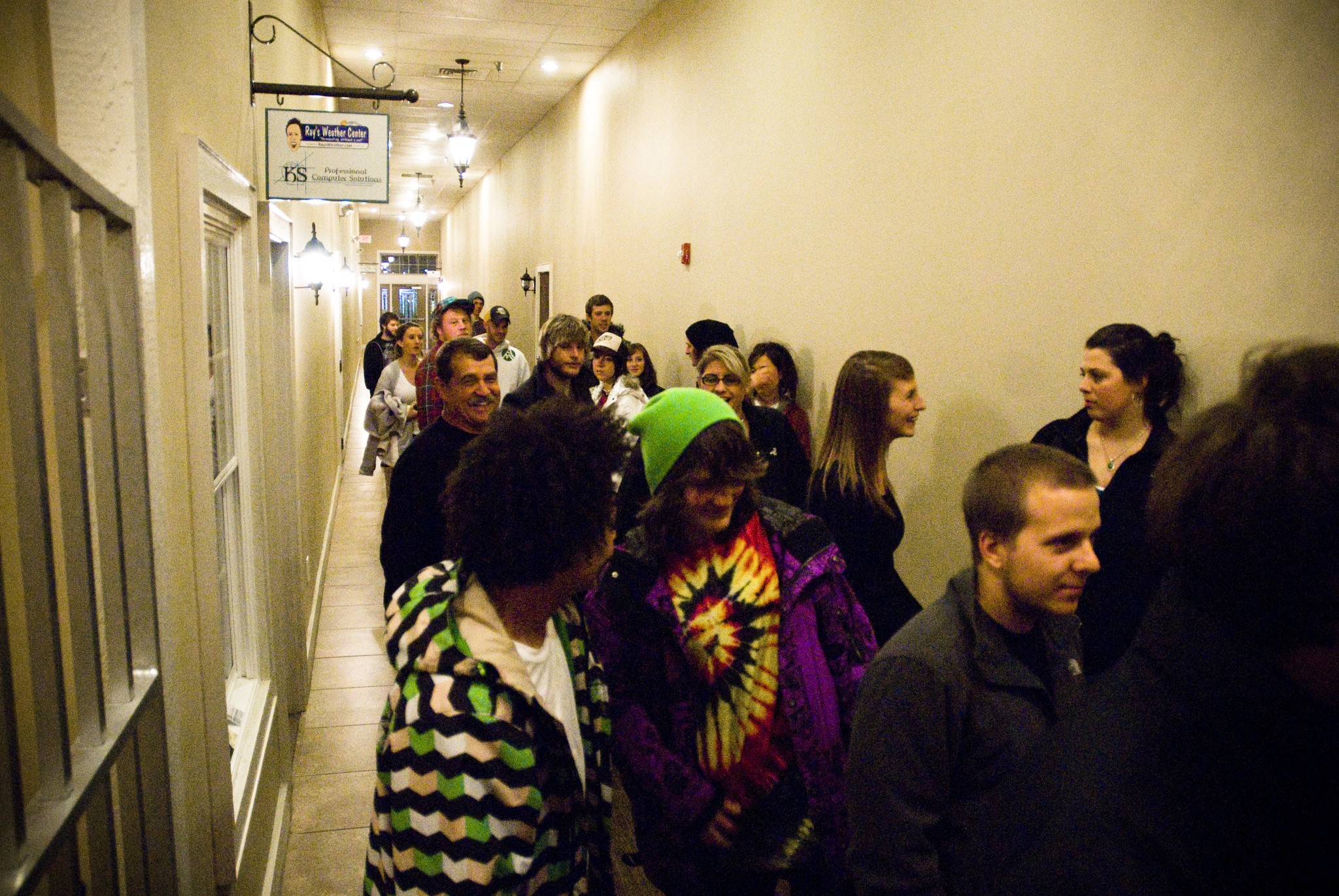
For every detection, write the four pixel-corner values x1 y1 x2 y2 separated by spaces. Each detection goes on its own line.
1102 418 1149 470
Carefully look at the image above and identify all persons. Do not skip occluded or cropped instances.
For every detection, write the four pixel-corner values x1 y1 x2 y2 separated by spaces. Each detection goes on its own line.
1032 338 1339 896
362 292 1201 896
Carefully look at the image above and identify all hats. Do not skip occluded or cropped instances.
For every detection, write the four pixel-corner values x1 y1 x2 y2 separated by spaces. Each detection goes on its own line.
626 387 747 497
484 306 511 325
468 291 484 304
590 332 627 358
431 298 475 323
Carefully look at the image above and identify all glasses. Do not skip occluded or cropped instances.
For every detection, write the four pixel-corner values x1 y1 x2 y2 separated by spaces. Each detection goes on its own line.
697 374 742 387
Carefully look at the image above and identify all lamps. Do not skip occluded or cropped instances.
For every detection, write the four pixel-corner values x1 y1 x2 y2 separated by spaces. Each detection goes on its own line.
294 223 333 306
398 173 427 253
339 204 354 219
333 258 355 297
447 59 478 187
520 268 536 296
